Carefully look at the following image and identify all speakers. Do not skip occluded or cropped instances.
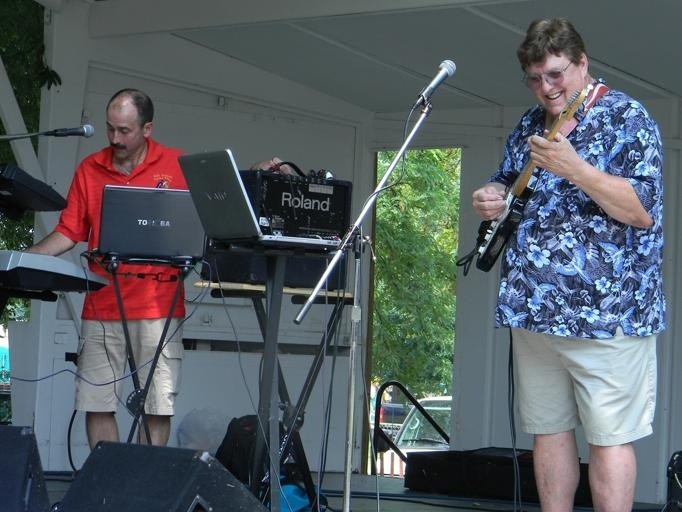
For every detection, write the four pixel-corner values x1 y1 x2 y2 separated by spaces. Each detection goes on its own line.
0 425 50 512
58 440 269 512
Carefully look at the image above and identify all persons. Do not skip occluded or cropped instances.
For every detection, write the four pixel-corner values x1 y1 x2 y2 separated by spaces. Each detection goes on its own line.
471 19 665 512
23 90 190 452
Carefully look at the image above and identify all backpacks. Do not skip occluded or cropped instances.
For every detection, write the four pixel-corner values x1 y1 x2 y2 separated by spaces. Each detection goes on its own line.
214 414 311 512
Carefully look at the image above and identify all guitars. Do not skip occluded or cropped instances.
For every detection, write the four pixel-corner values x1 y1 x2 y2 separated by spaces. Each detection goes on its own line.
476 88 586 272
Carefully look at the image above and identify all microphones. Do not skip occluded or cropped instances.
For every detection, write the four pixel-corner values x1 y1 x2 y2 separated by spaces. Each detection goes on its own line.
412 59 457 110
45 124 94 138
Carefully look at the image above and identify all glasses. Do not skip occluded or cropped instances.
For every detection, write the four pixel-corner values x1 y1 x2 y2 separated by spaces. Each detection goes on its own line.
521 57 574 90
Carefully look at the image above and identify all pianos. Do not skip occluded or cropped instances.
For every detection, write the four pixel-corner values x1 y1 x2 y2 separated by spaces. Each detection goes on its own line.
0 250 109 300
0 164 67 219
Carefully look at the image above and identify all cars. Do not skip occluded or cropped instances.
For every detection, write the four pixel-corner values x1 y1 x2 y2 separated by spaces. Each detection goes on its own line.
377 396 452 478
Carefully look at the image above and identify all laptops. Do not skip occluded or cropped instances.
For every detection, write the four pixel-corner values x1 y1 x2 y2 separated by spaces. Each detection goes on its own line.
98 184 206 259
177 148 342 250
